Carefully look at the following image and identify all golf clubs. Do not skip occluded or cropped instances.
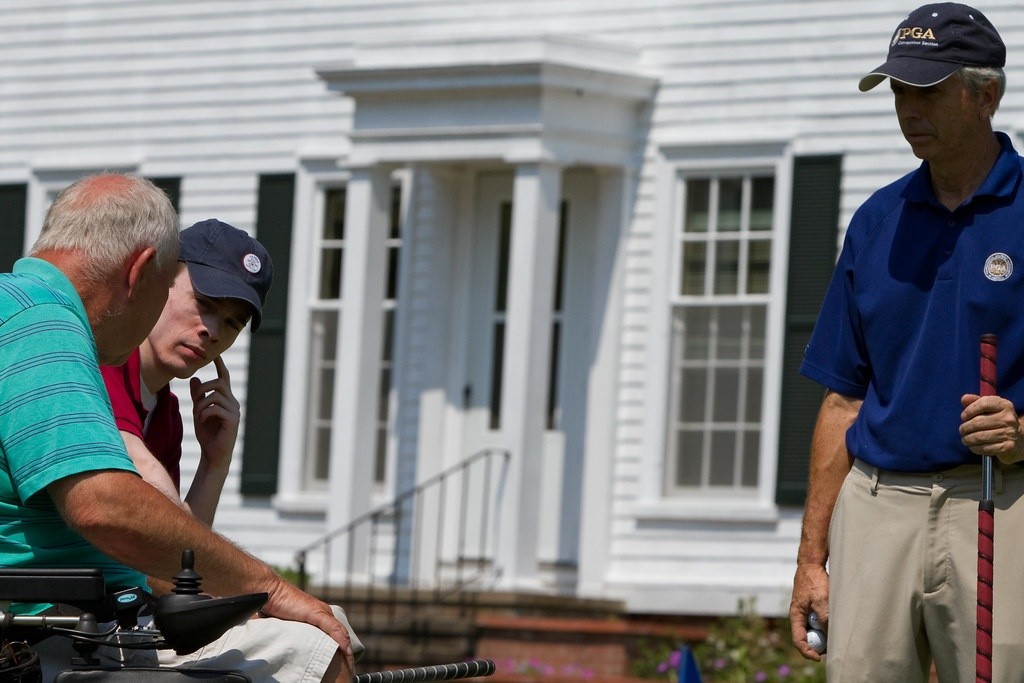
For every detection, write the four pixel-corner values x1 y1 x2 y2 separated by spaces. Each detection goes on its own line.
349 659 497 682
974 330 994 681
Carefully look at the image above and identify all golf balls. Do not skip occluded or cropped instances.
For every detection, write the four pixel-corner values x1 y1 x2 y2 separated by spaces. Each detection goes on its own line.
806 611 826 655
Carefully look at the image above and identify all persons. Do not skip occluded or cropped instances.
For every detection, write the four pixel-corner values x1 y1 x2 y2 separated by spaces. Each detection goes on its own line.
788 2 1023 683
0 168 357 683
98 217 271 613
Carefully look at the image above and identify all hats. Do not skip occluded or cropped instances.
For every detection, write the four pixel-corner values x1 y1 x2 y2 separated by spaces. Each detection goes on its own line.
858 2 1006 92
175 218 272 333
